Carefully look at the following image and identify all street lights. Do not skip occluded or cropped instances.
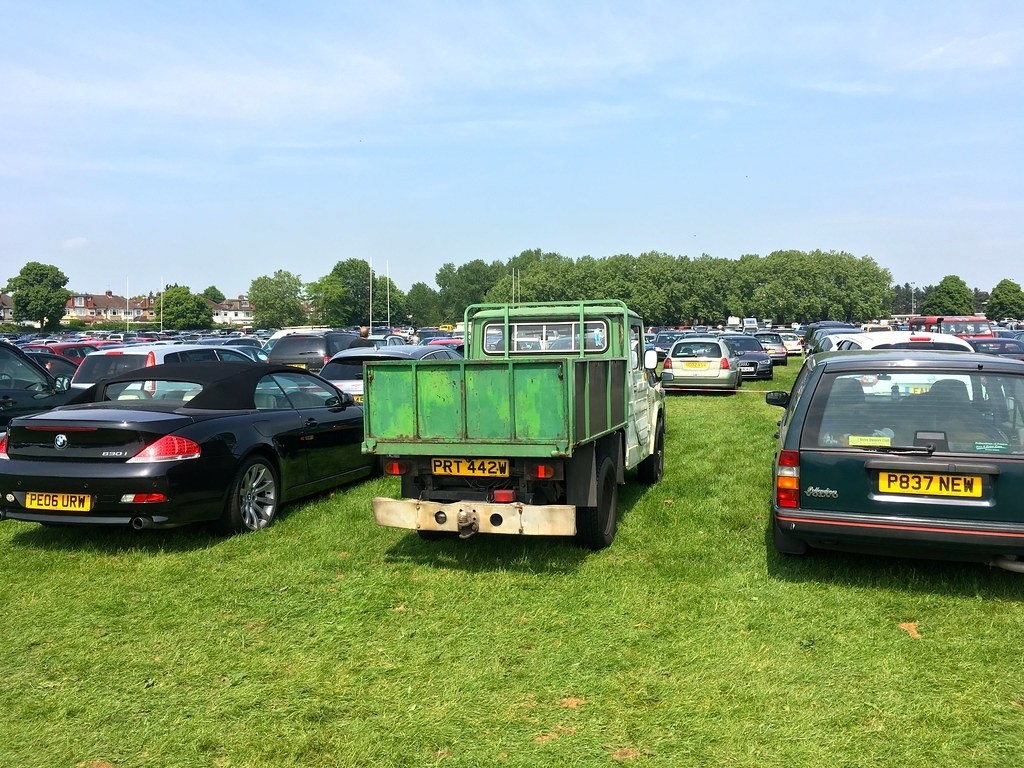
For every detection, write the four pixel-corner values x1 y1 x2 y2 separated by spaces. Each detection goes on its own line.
911 282 915 314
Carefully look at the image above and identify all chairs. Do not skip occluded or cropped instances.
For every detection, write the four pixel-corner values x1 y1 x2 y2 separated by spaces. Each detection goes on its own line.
495 340 521 350
254 394 277 408
927 379 971 424
682 348 693 354
118 389 202 401
822 378 867 407
577 337 603 350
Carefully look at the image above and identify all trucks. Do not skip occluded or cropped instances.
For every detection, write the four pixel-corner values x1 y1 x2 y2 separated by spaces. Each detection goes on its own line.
364 296 668 550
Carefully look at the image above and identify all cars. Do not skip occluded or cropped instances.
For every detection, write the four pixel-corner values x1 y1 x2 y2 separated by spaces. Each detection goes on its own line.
720 335 774 380
805 330 1005 404
343 323 605 355
0 340 70 431
307 342 464 410
754 333 788 364
0 362 381 534
0 326 275 380
639 314 968 366
766 350 1024 579
660 338 742 394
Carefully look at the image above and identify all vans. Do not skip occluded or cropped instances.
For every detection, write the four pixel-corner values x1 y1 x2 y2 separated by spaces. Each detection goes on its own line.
70 343 299 406
256 325 340 358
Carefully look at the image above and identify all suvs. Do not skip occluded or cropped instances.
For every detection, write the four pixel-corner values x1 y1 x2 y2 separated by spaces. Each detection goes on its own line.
909 316 1024 362
268 332 374 375
